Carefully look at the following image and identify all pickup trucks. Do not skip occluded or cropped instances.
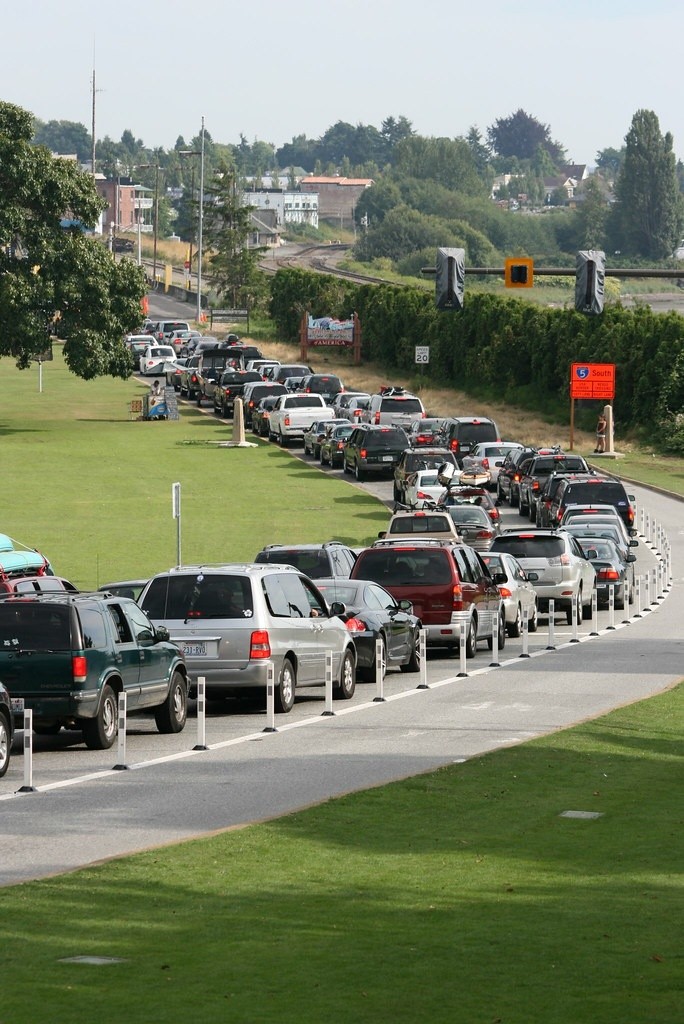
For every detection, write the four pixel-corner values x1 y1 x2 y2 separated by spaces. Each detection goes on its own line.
378 510 458 541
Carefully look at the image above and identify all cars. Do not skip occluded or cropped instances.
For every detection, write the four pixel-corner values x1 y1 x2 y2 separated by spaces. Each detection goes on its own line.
127 320 442 480
460 441 589 519
99 580 149 613
430 505 498 553
305 579 427 682
0 682 16 775
478 552 542 637
558 504 639 608
401 471 501 534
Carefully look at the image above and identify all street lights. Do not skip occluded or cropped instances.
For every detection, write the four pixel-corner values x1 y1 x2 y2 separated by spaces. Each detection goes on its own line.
181 151 203 324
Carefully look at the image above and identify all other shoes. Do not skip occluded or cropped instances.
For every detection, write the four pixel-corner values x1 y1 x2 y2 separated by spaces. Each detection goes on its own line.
594 449 598 453
598 450 604 453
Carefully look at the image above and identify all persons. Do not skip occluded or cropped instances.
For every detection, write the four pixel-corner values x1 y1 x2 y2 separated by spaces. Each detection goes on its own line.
594 413 607 453
152 381 160 395
312 609 319 617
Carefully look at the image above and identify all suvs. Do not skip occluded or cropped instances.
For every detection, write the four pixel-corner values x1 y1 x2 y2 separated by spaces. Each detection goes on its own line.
391 445 461 502
491 528 600 626
436 417 502 466
0 592 191 751
0 570 83 624
138 565 358 714
254 546 358 606
352 537 508 659
533 471 636 536
